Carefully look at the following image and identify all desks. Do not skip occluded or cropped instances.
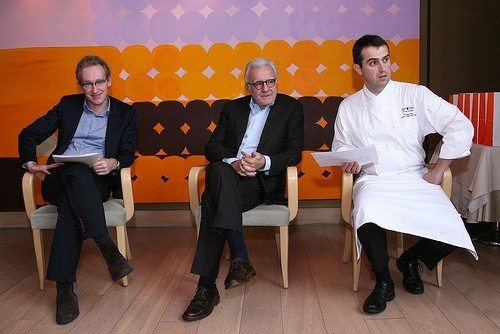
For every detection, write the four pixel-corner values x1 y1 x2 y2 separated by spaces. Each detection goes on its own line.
449 143 500 247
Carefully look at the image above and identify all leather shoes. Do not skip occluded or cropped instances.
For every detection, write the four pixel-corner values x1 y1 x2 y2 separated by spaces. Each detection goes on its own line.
364 278 395 313
182 283 220 320
225 260 257 289
56 289 79 323
107 252 133 281
395 251 424 293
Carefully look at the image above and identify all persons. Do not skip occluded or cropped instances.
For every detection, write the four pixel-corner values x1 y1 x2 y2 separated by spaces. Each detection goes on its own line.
18 55 135 325
331 34 480 313
182 58 303 322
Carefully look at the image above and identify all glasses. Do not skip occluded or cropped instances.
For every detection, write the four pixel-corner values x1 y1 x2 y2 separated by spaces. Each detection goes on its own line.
247 79 275 90
83 76 107 87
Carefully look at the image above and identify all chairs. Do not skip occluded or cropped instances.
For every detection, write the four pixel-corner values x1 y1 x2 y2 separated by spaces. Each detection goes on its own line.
187 164 299 289
22 163 134 291
341 163 452 291
